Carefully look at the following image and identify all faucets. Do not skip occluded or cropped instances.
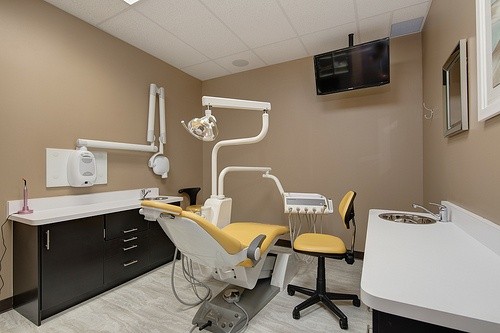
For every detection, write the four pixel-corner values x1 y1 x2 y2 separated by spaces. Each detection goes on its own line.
141 189 151 198
411 200 448 222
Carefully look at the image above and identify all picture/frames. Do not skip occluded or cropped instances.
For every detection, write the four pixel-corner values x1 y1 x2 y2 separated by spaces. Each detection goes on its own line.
442 39 469 137
476 0 500 124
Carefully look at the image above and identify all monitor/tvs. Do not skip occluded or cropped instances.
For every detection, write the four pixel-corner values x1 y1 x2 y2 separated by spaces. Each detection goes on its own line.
313 37 391 95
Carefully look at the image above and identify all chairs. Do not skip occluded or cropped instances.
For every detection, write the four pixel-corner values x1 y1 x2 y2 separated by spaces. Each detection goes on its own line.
139 200 297 291
287 191 360 330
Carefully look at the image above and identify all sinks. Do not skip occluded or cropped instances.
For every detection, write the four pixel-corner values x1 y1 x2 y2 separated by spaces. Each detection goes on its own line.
138 196 168 201
377 211 436 224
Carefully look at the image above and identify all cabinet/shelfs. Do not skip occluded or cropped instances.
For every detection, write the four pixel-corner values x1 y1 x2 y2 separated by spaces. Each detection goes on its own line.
12 202 181 327
